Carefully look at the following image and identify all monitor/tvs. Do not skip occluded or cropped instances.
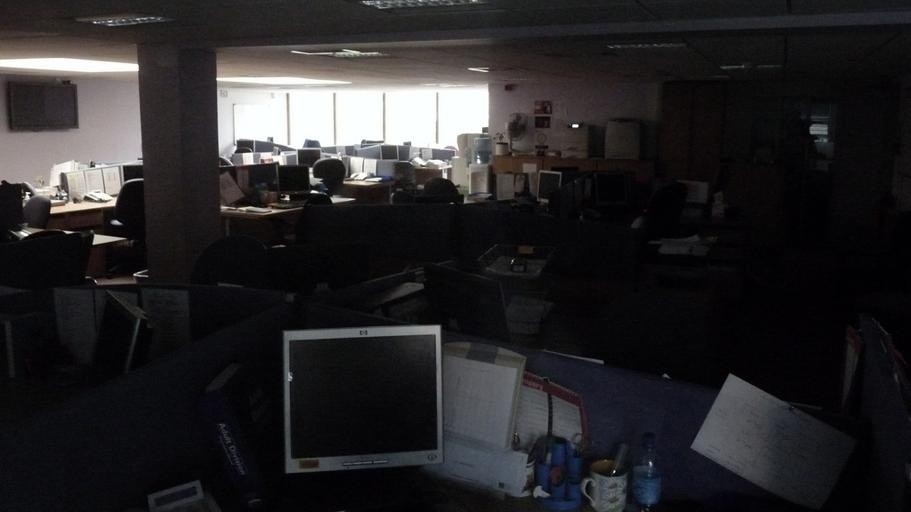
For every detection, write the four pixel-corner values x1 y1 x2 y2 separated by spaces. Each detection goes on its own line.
237 140 255 152
281 324 444 475
89 293 148 386
380 145 411 161
593 171 629 206
277 165 312 194
121 165 144 183
424 262 512 343
537 170 562 203
297 149 321 166
275 238 370 298
8 81 79 130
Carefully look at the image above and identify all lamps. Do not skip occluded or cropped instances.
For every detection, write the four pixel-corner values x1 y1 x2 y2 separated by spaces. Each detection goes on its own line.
360 0 482 8
78 11 177 29
721 63 782 71
290 48 391 58
608 39 691 50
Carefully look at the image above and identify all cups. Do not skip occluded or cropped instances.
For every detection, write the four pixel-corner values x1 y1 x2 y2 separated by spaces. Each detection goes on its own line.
579 458 629 512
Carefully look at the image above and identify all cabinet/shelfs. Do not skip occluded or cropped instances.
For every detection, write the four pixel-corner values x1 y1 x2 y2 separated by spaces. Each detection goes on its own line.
663 80 728 183
832 82 899 195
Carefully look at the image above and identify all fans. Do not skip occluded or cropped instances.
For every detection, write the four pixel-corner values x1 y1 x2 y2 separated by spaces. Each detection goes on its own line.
505 112 530 154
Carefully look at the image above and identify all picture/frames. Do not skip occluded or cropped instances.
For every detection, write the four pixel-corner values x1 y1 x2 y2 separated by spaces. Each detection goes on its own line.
534 99 553 129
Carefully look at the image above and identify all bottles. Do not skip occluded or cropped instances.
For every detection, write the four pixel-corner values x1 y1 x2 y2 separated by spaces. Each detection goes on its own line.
631 431 664 512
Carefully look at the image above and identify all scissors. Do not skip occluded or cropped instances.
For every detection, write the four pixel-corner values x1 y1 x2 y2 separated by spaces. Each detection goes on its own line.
571 433 594 458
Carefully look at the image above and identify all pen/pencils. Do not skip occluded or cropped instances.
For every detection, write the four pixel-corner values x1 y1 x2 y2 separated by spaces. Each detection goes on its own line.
532 435 567 472
